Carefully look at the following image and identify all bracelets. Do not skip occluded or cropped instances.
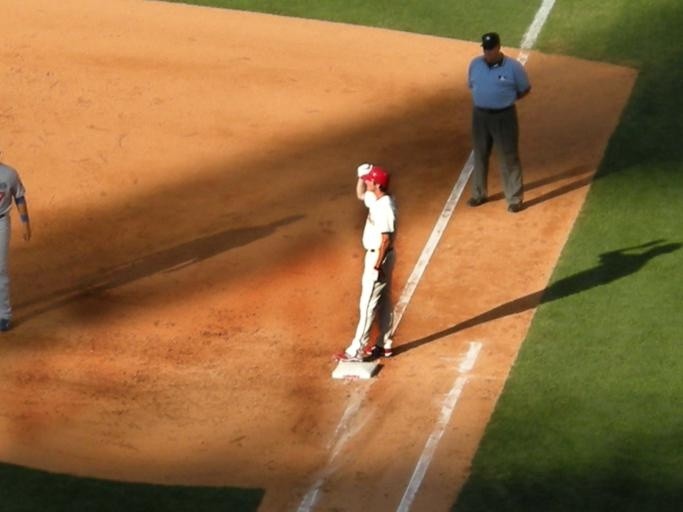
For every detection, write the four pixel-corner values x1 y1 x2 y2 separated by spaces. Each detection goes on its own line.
19 213 27 223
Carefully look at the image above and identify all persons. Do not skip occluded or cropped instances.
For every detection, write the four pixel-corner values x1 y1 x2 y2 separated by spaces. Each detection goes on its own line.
328 164 397 360
0 161 31 333
466 33 531 213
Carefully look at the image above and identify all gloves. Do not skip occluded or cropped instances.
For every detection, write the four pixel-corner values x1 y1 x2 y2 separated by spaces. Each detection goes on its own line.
357 162 373 178
371 266 380 283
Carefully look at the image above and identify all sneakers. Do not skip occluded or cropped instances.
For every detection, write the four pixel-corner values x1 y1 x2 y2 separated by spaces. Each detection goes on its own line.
1 318 10 332
467 196 486 206
365 342 392 357
332 347 368 363
508 202 524 212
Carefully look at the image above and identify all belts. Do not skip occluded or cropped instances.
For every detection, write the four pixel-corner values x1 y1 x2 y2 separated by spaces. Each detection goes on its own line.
476 105 514 113
366 246 394 253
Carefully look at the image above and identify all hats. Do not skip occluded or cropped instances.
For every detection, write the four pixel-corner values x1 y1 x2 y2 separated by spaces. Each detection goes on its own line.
361 167 390 192
481 31 500 49
